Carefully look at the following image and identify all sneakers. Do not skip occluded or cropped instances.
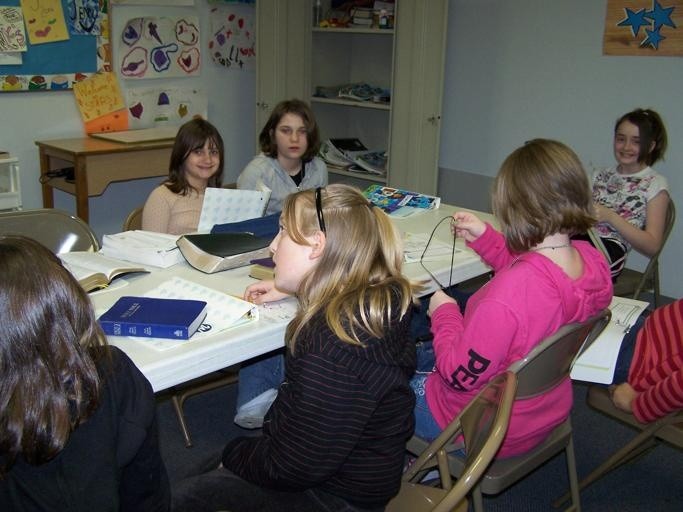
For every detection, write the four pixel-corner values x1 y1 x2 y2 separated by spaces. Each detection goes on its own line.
233 387 280 430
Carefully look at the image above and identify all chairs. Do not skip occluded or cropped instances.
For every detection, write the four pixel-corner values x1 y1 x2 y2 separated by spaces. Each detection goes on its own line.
551 380 682 511
402 303 616 511
608 198 675 309
119 199 255 447
0 210 103 268
370 370 520 511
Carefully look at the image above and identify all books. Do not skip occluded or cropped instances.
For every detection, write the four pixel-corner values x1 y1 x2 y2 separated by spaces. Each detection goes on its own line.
195 178 271 232
122 276 260 351
398 230 468 265
349 9 374 19
99 229 184 269
352 17 376 27
95 295 206 341
176 231 272 274
55 251 151 297
322 136 385 178
361 184 440 221
247 256 277 282
569 285 650 388
346 23 374 30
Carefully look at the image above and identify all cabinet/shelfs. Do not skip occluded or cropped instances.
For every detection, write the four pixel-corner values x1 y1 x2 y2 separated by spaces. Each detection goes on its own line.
286 1 445 195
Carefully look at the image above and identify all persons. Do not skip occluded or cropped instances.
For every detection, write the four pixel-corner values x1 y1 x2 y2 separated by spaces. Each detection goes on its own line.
0 232 173 512
168 184 422 511
235 99 328 217
572 107 670 282
140 118 287 430
406 138 614 487
406 285 681 424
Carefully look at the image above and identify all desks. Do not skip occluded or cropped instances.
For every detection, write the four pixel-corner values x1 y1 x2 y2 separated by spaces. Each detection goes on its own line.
35 135 226 234
63 186 509 409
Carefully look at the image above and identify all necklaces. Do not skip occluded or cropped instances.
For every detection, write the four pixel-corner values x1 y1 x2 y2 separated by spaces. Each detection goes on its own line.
509 242 570 268
279 159 303 189
